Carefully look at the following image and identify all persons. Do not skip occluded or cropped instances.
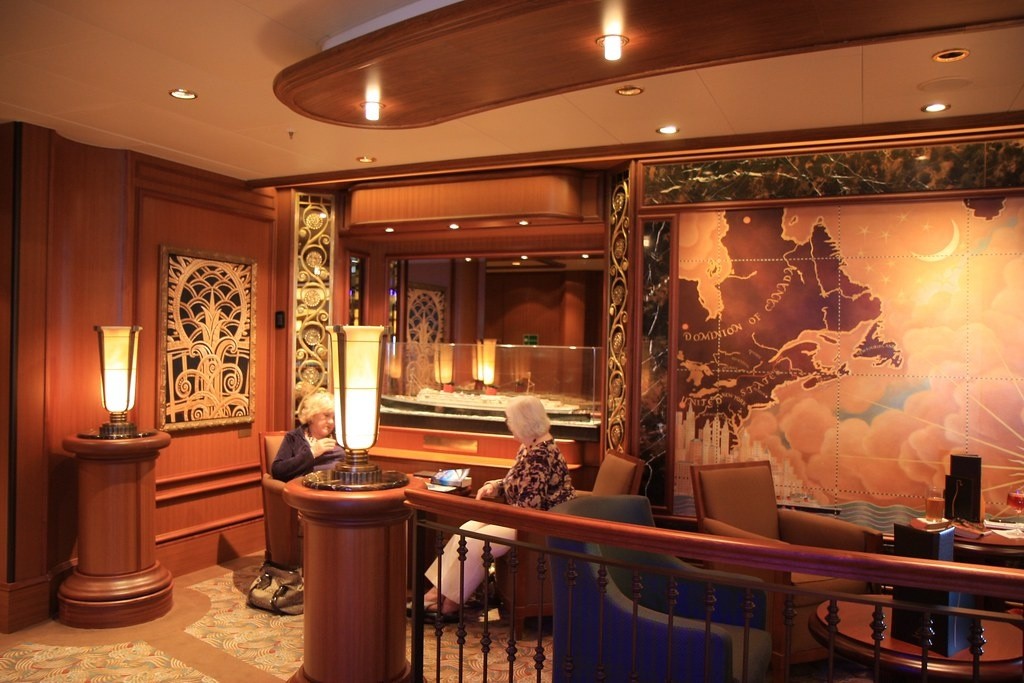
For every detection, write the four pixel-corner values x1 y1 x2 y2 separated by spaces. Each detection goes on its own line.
271 392 345 483
406 396 575 621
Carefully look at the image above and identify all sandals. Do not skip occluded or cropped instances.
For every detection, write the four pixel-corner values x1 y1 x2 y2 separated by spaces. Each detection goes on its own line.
424 615 459 624
407 600 443 619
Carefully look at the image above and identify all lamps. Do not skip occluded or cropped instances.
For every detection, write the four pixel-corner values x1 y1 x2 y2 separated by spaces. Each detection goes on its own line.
76 325 158 440
433 337 501 396
302 324 410 492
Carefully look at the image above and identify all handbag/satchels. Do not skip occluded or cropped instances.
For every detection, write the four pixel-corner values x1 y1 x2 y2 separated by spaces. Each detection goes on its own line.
247 561 304 615
462 574 497 608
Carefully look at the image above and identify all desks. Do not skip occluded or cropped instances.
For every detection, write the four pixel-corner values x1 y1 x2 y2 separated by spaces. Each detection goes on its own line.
402 472 474 605
908 515 1024 613
807 592 1024 683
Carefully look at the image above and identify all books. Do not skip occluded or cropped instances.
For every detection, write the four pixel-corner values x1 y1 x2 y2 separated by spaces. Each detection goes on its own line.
425 468 473 492
910 517 1024 540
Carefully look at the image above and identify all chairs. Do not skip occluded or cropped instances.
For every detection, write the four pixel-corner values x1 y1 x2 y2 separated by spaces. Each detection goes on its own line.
689 459 885 683
493 448 646 641
542 494 774 683
257 430 305 572
